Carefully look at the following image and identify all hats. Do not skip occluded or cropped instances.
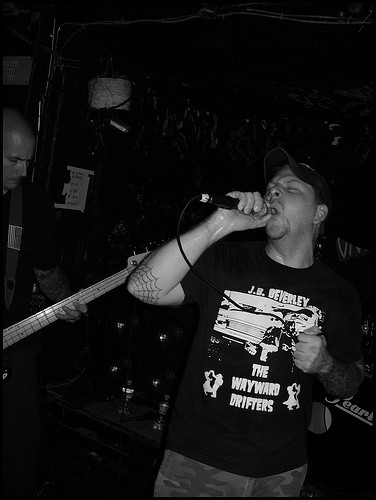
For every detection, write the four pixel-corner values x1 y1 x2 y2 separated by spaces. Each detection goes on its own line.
263 148 332 212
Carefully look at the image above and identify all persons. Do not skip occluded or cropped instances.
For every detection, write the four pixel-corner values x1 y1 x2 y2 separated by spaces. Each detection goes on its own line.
127 147 365 500
0 108 89 499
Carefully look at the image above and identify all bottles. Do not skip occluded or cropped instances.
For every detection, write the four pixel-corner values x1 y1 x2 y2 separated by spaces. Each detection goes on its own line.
117 380 135 416
152 394 170 433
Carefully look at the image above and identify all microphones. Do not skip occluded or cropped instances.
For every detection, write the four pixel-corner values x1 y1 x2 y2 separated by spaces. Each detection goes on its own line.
199 194 268 219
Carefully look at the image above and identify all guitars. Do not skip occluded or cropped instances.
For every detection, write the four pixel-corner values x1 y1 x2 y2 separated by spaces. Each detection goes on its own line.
2 247 153 351
